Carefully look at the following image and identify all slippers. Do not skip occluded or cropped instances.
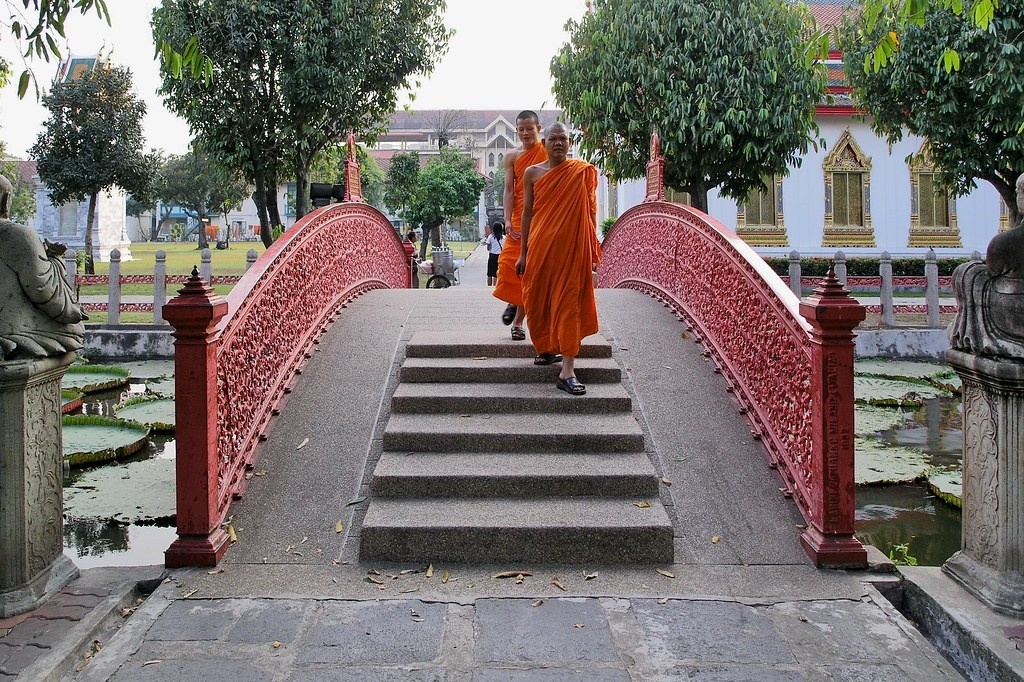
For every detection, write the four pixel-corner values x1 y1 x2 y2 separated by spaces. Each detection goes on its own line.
556 377 586 395
534 353 563 365
503 304 517 326
511 327 526 341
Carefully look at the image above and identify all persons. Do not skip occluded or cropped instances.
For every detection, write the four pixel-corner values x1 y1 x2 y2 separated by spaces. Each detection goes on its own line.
492 110 548 341
486 218 506 286
407 232 421 288
515 122 601 395
947 173 1024 360
0 174 90 336
